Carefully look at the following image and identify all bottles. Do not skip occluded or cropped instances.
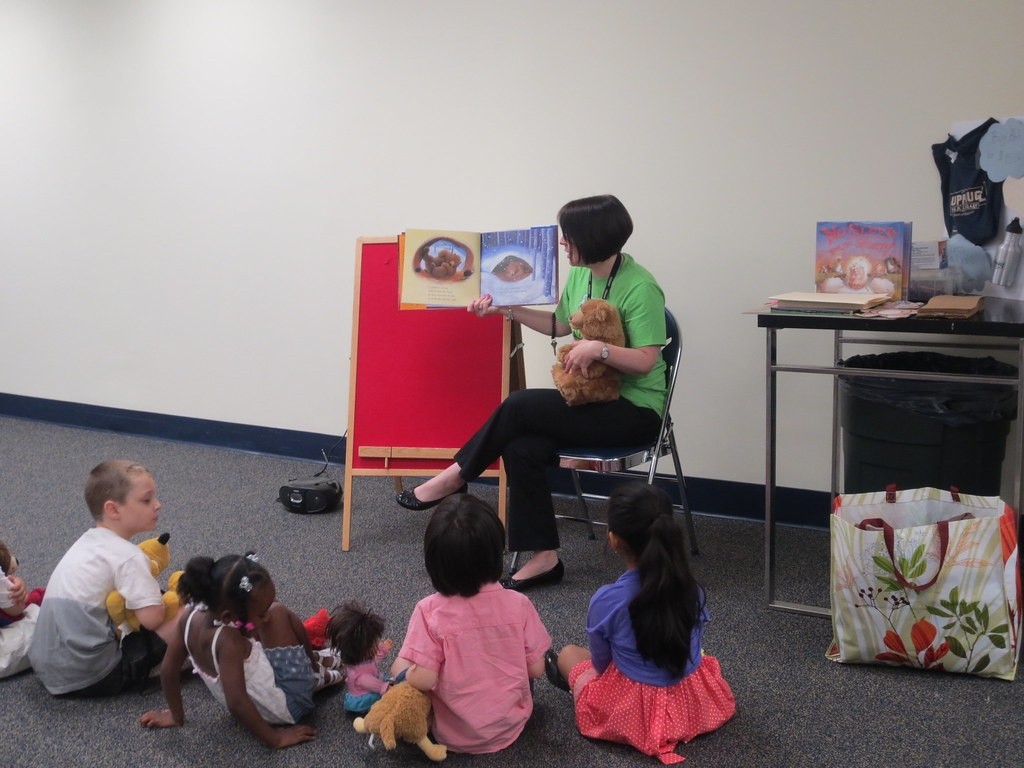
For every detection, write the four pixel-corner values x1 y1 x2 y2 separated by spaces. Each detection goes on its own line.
992 216 1023 287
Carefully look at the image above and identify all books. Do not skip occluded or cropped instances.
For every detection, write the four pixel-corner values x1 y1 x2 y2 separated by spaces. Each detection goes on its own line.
769 219 987 318
400 225 561 312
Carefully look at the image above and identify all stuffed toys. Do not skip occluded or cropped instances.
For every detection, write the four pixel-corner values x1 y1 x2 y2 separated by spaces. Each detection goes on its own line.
327 605 393 715
105 532 187 666
551 299 625 406
0 541 46 627
353 681 448 762
304 609 331 649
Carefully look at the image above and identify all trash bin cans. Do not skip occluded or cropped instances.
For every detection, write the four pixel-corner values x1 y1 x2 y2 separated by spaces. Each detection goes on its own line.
837 352 1019 497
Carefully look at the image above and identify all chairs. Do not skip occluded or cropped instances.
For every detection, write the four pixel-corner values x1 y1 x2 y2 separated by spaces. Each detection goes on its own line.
508 309 699 574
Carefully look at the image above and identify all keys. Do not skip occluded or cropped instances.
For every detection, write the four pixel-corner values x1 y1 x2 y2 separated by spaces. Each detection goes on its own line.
551 340 558 356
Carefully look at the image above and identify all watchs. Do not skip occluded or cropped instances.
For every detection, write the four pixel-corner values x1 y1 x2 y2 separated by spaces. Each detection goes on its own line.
601 342 608 362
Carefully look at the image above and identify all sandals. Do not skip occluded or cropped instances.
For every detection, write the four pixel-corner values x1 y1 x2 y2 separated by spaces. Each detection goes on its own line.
311 647 348 693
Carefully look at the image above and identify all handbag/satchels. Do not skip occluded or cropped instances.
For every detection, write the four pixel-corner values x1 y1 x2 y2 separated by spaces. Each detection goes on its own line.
823 483 1022 683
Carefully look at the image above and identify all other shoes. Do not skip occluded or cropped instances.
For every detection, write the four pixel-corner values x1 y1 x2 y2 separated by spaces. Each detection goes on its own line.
544 648 574 697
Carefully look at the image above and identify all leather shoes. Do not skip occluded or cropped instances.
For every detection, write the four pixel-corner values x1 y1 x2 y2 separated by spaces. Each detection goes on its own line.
397 482 470 511
500 556 565 592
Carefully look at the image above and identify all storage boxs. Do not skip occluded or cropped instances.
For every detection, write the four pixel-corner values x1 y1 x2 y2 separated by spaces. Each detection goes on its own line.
909 267 964 301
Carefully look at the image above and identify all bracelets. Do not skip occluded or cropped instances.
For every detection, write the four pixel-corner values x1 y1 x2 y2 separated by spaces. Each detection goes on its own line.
505 304 513 322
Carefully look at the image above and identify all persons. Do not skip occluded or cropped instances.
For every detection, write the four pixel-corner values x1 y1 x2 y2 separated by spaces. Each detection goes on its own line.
392 496 552 756
0 567 40 679
29 461 184 698
547 483 734 755
396 194 667 590
139 552 344 748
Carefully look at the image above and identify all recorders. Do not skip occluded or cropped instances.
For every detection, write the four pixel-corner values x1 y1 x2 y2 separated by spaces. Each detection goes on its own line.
279 449 343 515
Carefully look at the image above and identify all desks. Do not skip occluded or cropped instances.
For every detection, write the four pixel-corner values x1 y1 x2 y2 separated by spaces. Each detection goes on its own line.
756 296 1024 617
